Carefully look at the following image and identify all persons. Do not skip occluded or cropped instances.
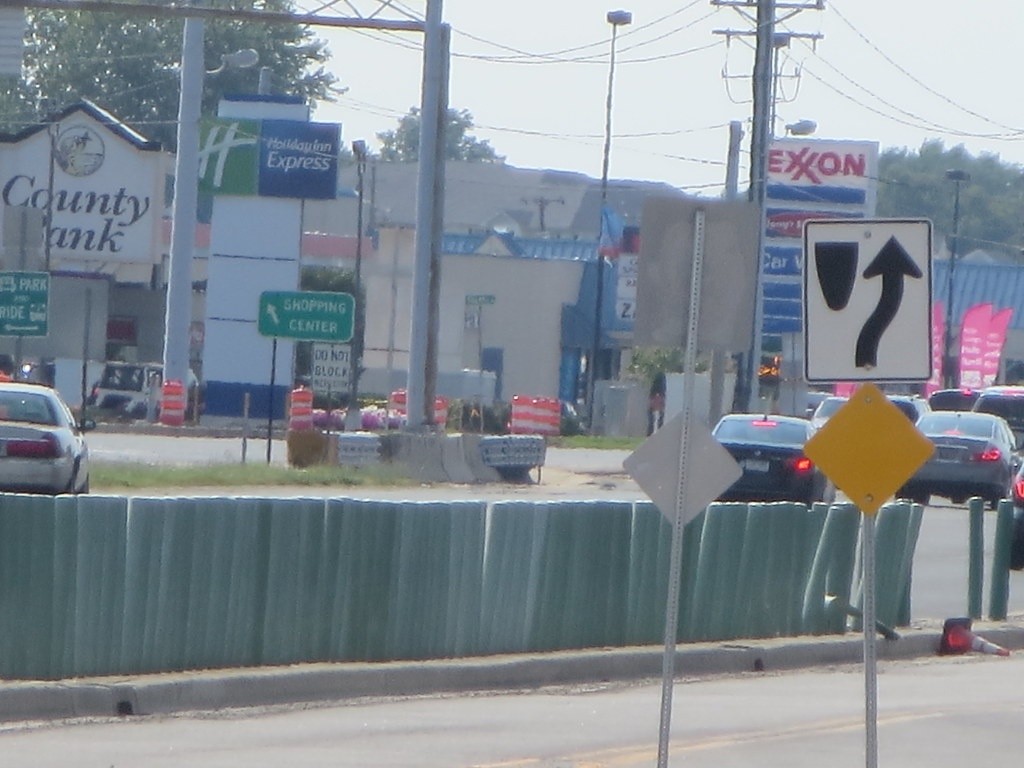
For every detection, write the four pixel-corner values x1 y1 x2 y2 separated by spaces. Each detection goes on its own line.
647 372 667 436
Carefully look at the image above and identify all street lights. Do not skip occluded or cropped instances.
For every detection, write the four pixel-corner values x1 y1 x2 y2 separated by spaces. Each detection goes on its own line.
345 140 368 413
581 8 632 435
939 168 972 388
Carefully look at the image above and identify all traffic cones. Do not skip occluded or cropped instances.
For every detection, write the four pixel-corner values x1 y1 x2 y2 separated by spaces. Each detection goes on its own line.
939 617 1013 659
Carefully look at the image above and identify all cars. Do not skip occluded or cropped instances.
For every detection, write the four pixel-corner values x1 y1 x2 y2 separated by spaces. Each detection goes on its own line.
706 411 835 507
0 380 97 494
808 385 1024 571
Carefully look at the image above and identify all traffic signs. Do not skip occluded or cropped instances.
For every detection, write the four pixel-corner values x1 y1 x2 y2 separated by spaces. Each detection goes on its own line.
0 270 51 339
257 290 356 344
